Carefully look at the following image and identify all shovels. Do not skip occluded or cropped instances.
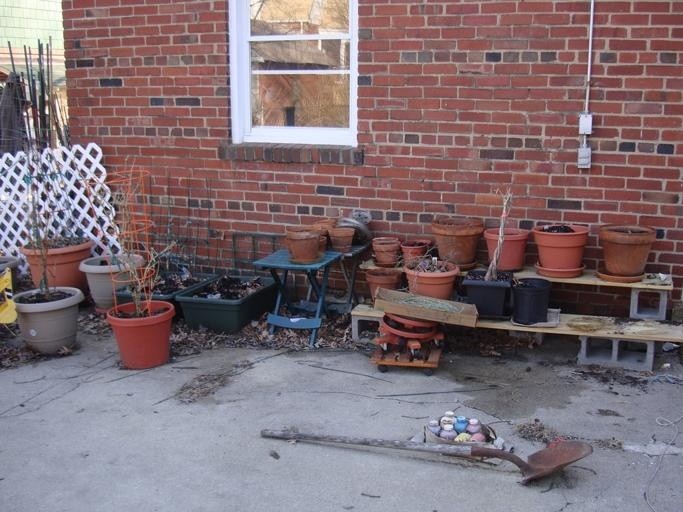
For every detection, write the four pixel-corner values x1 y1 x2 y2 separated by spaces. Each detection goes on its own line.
261 430 594 484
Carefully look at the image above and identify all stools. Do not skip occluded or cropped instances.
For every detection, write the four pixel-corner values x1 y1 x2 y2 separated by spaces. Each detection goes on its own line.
300 239 370 320
254 249 341 347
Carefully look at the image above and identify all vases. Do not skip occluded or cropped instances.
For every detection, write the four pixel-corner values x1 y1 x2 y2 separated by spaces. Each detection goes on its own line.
366 218 657 324
11 236 274 369
285 219 355 264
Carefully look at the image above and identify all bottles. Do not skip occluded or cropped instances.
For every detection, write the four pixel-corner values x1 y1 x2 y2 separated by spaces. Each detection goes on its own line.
428 411 486 446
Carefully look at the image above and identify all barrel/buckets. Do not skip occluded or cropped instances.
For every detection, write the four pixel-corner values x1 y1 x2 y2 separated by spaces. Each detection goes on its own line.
511 278 551 323
